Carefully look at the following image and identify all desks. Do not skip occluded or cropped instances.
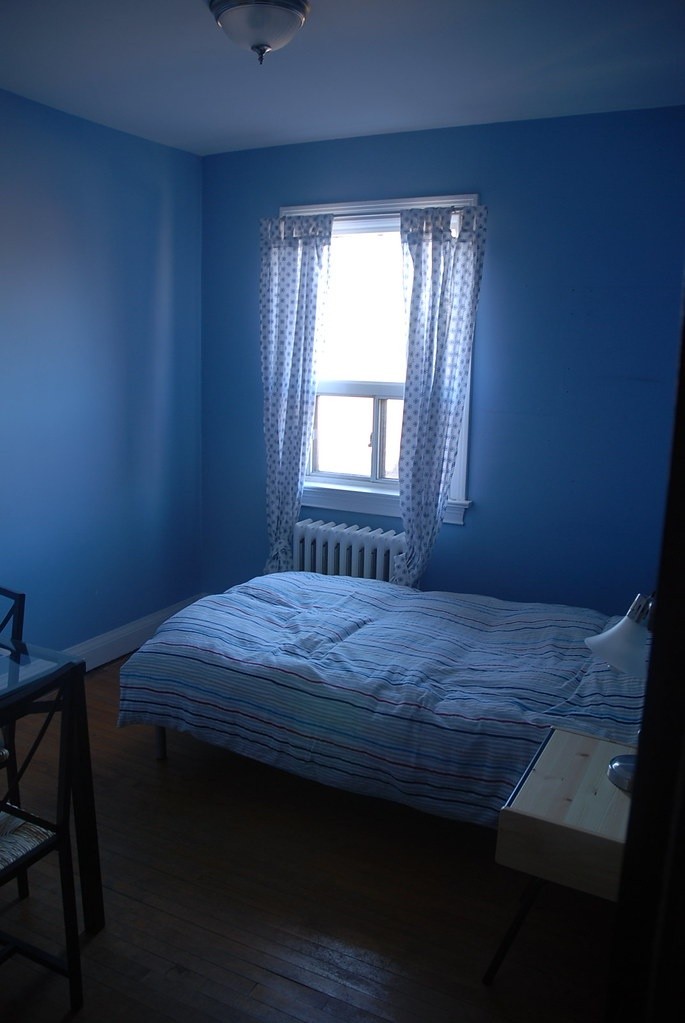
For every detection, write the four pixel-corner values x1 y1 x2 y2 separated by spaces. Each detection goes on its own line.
0 636 106 930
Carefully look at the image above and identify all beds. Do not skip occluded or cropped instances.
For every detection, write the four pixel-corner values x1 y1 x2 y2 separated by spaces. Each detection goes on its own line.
117 571 658 829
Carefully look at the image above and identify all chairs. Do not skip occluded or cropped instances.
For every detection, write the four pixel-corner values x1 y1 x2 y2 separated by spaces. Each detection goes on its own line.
0 585 26 806
0 661 85 1015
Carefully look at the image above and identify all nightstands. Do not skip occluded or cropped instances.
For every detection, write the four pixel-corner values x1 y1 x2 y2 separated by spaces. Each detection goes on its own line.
481 725 639 987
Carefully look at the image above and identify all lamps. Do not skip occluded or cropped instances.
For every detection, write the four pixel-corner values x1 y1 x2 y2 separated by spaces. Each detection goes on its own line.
584 593 653 792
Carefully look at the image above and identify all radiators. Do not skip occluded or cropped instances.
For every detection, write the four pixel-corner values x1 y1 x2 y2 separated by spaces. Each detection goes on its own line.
293 518 408 583
